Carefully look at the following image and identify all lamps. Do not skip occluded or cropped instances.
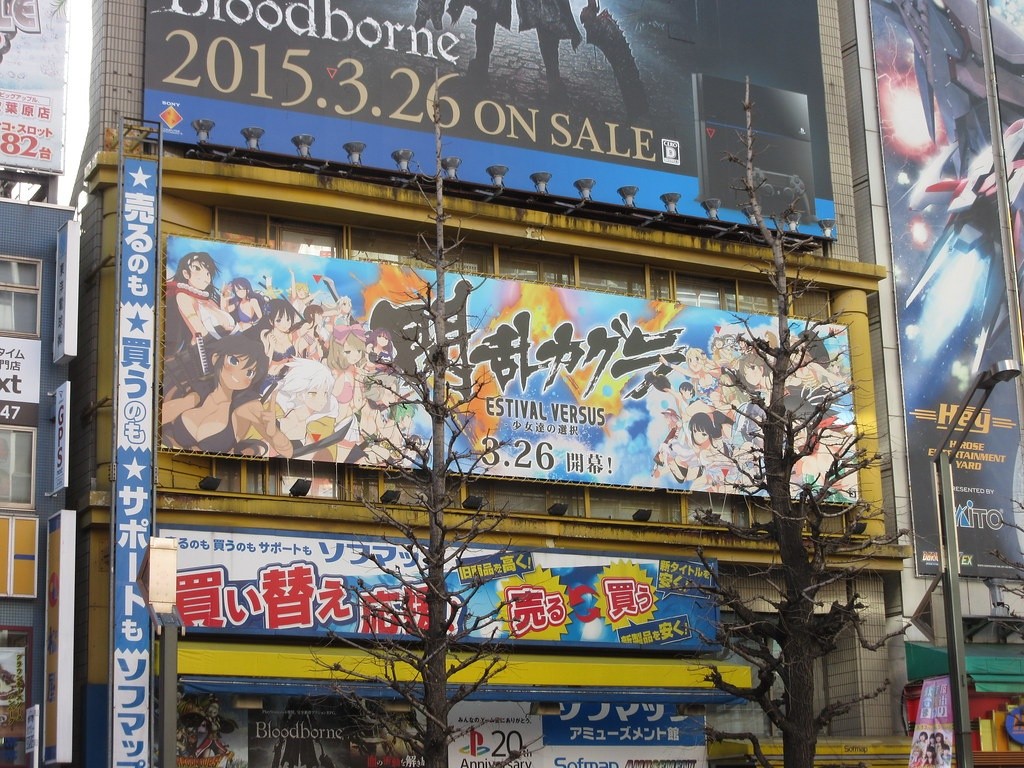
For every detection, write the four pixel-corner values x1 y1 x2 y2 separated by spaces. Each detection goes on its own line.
198 476 221 490
241 127 265 150
190 120 215 145
380 490 401 504
617 186 639 207
573 179 596 200
343 142 366 166
441 157 462 180
290 134 315 158
701 199 835 238
290 478 312 497
548 503 568 516
391 150 414 173
850 523 867 534
462 496 484 510
529 172 552 194
633 508 652 522
702 514 721 526
487 165 510 187
660 193 681 213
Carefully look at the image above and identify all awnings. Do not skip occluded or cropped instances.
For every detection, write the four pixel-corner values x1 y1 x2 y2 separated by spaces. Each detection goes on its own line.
154 641 752 687
905 641 1024 693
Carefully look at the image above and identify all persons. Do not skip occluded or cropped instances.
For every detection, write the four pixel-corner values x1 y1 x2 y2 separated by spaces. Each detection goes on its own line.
909 732 951 768
